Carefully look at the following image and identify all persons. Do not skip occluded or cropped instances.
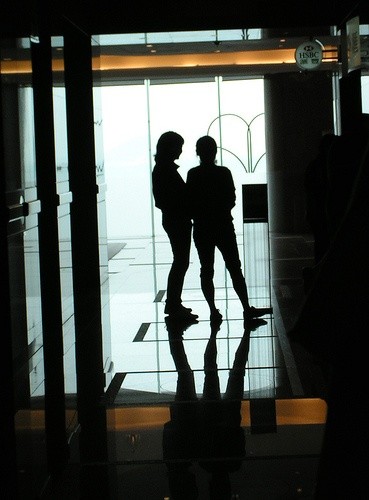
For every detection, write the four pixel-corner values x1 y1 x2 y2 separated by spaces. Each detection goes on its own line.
153 132 192 315
186 136 271 320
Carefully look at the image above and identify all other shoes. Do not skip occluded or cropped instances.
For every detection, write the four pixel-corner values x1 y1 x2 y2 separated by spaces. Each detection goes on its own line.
166 321 198 332
164 304 192 313
244 307 265 318
211 321 222 331
244 319 267 332
169 313 198 319
210 312 222 322
